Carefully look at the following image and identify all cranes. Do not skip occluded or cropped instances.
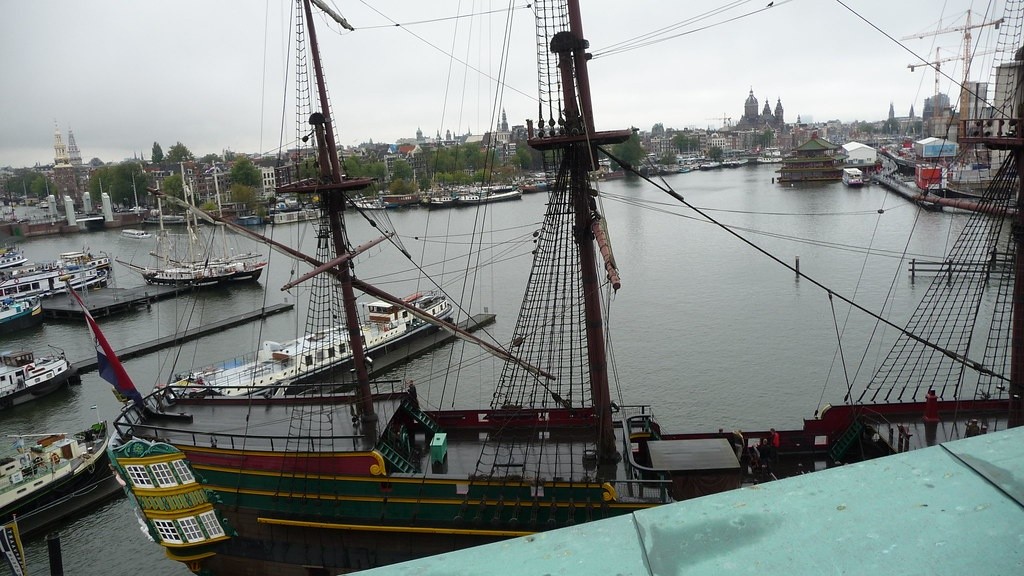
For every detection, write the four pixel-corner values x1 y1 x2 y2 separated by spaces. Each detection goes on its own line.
899 8 1010 149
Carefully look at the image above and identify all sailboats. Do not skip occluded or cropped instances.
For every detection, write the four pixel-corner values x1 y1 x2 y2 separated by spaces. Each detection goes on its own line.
114 160 268 286
107 1 1024 575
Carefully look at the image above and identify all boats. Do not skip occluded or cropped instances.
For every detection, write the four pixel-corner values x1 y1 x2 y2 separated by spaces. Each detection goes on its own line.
0 242 113 336
842 168 864 187
152 292 455 399
756 157 783 164
602 156 749 181
271 167 603 225
0 403 111 528
0 342 79 413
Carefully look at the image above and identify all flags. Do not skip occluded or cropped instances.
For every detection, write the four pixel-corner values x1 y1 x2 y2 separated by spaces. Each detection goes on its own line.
66 280 144 402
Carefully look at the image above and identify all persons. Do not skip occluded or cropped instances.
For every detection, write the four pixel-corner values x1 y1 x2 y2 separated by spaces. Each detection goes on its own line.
406 380 421 411
733 429 779 483
